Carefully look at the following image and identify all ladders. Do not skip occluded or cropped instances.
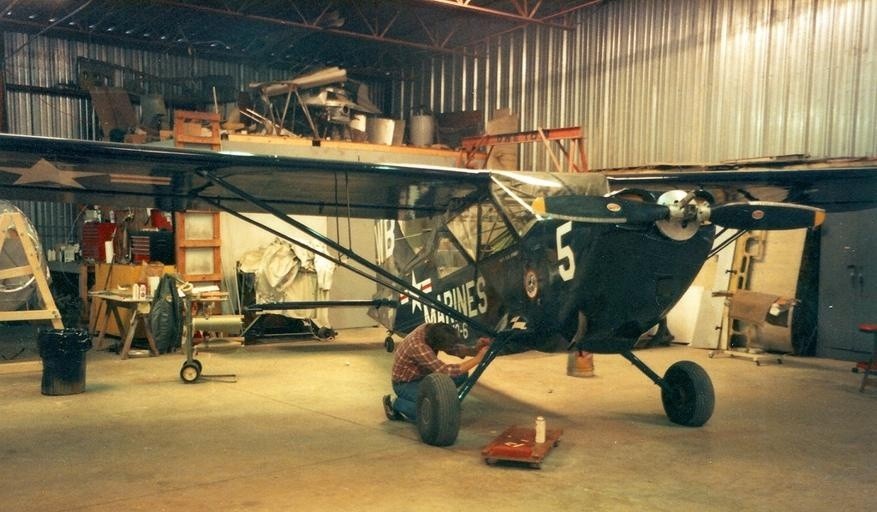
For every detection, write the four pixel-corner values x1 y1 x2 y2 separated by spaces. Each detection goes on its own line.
172 111 221 342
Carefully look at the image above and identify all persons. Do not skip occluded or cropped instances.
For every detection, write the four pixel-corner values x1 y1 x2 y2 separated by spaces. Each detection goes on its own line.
382 321 496 422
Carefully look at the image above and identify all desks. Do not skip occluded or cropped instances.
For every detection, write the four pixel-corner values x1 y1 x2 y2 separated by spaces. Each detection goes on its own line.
851 323 877 392
47 260 96 321
91 288 230 359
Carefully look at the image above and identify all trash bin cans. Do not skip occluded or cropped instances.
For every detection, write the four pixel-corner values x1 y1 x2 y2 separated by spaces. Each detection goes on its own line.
38 328 89 396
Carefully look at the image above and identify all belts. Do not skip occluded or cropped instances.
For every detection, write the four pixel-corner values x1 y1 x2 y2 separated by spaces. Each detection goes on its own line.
392 379 421 385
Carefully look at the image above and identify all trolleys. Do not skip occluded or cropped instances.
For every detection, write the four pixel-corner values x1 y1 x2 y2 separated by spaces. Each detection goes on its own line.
163 272 240 383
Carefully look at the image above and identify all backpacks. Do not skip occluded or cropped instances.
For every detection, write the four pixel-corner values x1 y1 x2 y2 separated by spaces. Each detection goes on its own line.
147 272 184 355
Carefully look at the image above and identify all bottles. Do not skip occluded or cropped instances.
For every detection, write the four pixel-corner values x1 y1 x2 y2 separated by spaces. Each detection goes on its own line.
133 283 147 300
535 416 547 444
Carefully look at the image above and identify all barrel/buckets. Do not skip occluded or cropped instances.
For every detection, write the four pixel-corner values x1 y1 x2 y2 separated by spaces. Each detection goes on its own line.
366 117 406 147
410 115 434 148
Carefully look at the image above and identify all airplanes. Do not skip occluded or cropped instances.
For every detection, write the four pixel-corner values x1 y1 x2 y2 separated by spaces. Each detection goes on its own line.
0 130 876 447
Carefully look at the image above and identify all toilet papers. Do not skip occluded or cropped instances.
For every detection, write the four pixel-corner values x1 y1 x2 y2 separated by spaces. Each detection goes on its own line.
105 241 115 264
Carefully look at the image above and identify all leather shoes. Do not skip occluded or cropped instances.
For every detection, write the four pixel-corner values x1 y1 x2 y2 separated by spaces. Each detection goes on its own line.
382 394 405 421
